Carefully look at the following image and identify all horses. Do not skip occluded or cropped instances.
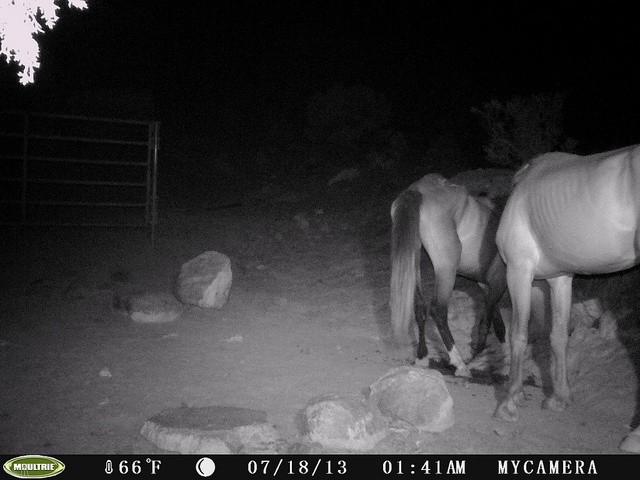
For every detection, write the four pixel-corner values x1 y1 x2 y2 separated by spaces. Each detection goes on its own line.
495 141 640 455
386 169 515 382
